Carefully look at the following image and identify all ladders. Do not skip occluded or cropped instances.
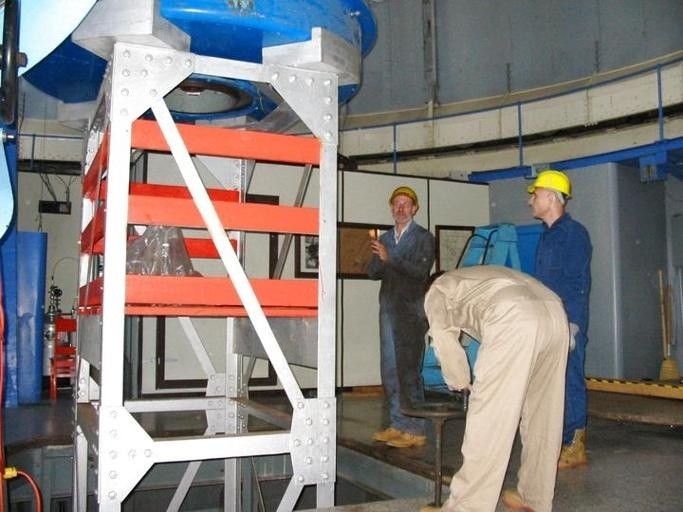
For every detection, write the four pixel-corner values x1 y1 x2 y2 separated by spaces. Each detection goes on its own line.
421 222 522 391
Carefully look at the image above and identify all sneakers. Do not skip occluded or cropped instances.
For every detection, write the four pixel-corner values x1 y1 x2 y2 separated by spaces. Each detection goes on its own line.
503 488 532 511
374 427 427 447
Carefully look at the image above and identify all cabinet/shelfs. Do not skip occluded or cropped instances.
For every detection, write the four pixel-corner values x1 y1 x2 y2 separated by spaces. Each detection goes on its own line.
70 42 338 512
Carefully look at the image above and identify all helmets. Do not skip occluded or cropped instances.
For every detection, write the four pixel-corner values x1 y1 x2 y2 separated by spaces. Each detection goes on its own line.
388 187 418 215
526 171 571 199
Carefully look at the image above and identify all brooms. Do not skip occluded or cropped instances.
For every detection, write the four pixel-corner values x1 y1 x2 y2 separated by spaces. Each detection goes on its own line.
659 284 681 383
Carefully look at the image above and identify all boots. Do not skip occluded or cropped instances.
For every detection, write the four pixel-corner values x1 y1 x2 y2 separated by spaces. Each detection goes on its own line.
557 428 587 469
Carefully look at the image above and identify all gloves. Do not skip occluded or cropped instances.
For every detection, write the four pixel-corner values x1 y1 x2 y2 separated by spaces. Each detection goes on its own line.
569 322 579 352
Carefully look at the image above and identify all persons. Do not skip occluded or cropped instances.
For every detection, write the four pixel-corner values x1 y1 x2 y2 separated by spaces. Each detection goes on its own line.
524 170 592 473
420 264 571 512
364 187 436 448
